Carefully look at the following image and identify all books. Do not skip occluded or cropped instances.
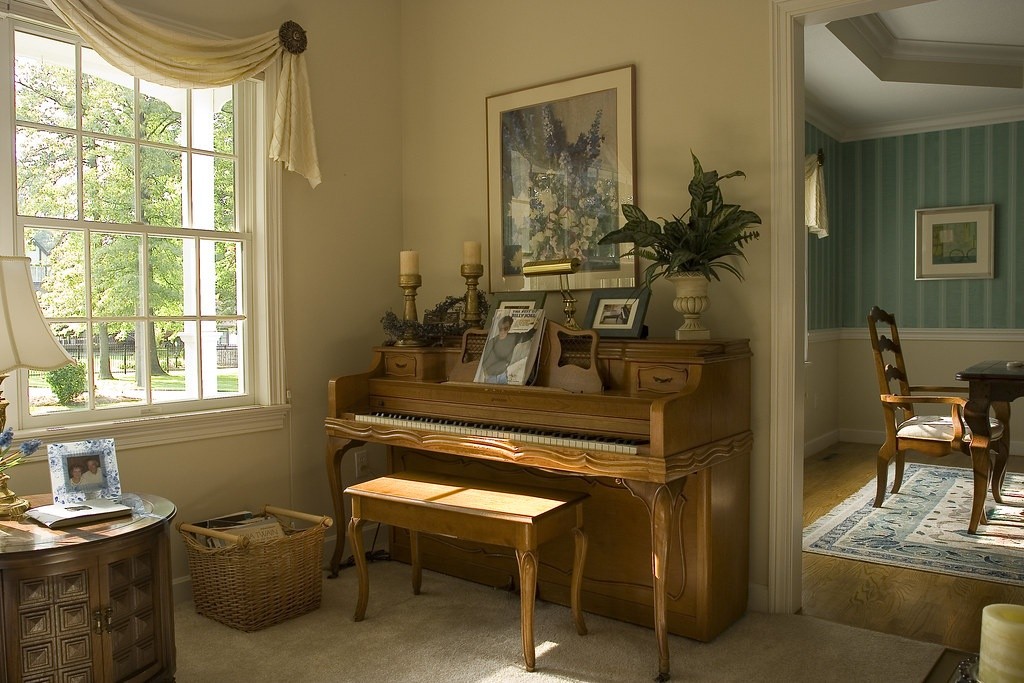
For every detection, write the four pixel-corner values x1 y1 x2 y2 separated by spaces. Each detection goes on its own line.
473 308 548 389
188 511 285 549
25 498 131 529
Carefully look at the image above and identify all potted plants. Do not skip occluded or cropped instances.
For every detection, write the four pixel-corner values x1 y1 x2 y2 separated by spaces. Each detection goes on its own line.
596 149 762 342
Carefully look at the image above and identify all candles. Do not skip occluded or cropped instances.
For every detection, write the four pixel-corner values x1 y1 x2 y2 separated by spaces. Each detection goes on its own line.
979 603 1024 683
400 250 420 276
463 240 482 265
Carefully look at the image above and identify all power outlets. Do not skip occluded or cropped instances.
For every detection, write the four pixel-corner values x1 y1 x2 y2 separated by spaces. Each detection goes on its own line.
355 451 370 477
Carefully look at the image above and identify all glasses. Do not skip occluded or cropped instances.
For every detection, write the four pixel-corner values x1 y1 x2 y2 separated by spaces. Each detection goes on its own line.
499 327 508 331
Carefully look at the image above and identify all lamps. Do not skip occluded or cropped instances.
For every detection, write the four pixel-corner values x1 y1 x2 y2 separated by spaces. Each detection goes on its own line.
522 256 582 331
0 256 77 519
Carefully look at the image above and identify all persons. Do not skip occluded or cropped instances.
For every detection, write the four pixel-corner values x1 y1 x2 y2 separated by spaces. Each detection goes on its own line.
69 459 103 484
620 307 628 324
483 315 537 384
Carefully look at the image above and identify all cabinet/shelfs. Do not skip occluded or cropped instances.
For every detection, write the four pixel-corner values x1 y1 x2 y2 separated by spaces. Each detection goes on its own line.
0 492 177 683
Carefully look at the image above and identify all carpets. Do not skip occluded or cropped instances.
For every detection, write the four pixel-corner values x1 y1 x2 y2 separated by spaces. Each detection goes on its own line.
802 461 1024 586
172 558 949 683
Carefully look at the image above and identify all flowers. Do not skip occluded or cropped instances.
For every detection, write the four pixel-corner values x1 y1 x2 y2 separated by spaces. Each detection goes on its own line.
0 426 42 472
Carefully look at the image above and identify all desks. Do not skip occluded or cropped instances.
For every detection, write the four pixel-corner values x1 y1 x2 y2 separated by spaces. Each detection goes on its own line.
956 359 1024 534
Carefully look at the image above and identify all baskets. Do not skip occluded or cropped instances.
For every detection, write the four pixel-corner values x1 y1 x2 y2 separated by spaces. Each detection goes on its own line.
175 503 334 633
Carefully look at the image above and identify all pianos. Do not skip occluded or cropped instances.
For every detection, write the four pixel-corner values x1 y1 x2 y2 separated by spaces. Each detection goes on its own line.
324 338 754 682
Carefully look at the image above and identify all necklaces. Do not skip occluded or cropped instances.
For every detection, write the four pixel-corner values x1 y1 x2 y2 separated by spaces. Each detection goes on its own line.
71 478 82 484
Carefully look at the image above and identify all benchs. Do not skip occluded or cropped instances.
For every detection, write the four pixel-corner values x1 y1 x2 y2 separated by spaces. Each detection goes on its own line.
343 471 589 671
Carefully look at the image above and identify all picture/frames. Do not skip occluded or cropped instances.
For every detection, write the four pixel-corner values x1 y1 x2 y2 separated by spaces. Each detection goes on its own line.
47 437 122 505
484 292 547 331
581 287 652 337
914 203 996 282
484 64 637 294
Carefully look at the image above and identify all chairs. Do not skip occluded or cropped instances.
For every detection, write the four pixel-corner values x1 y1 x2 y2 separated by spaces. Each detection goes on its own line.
867 305 1008 526
